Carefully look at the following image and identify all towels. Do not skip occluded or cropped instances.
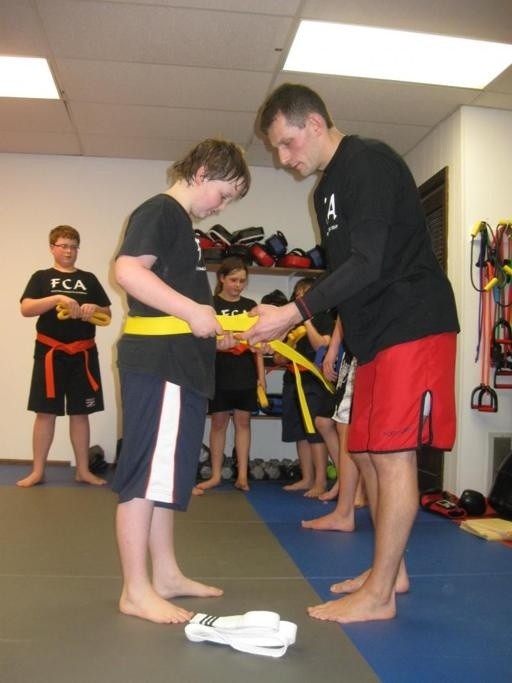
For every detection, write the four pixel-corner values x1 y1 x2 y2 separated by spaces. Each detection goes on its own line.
458 517 511 543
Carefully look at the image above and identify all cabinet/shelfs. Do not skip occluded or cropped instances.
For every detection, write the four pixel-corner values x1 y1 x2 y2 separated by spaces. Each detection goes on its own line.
199 262 335 419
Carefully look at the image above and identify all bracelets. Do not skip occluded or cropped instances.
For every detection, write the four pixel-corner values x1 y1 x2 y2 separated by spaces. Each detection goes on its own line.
294 297 311 323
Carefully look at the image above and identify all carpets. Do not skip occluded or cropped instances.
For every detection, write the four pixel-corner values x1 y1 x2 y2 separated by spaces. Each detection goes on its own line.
2 480 379 683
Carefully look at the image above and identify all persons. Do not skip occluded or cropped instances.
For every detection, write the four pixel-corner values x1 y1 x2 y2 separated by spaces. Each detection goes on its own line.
108 159 206 497
235 83 458 622
15 224 112 490
113 137 249 624
196 255 266 492
272 275 331 499
294 317 360 534
314 314 367 509
